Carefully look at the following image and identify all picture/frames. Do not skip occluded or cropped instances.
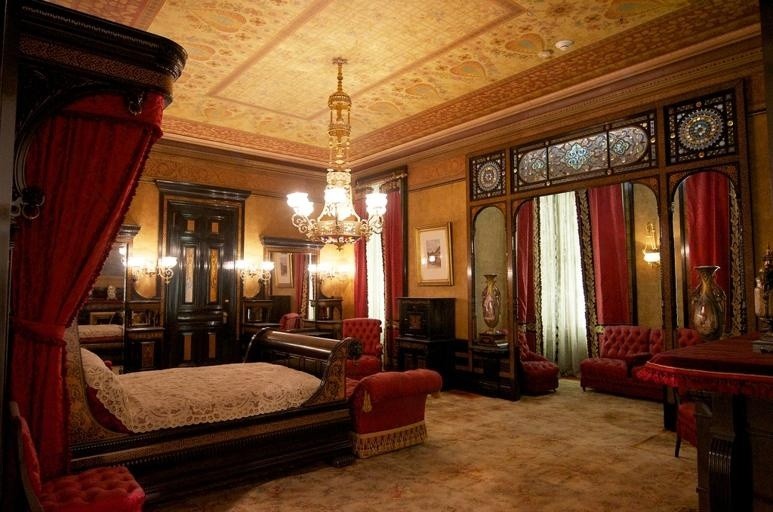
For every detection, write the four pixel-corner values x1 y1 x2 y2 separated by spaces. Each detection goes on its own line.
414 221 454 287
272 249 295 289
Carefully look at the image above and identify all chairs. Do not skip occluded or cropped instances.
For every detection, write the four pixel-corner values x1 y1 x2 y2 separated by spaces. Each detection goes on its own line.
8 398 151 512
341 317 384 385
277 310 303 330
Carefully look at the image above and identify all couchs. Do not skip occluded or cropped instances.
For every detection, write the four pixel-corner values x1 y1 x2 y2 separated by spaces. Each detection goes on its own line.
341 367 443 459
580 324 700 406
501 326 561 396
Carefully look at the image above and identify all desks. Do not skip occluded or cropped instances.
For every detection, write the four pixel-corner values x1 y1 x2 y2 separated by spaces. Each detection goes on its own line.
630 329 773 512
80 325 167 371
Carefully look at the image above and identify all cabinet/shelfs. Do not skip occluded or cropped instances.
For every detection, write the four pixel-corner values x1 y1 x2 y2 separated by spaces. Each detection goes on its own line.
397 295 457 383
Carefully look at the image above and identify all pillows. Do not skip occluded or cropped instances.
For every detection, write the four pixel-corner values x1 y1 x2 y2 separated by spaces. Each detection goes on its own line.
79 343 132 432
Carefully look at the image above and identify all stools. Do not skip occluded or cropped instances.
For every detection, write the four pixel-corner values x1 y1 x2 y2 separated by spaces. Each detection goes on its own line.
667 382 700 458
76 323 126 376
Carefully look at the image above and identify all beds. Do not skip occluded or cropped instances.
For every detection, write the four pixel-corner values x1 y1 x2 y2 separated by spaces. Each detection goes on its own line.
51 324 364 506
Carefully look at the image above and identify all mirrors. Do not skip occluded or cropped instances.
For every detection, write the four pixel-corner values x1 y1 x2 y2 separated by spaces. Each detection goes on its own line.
76 222 141 326
262 234 325 330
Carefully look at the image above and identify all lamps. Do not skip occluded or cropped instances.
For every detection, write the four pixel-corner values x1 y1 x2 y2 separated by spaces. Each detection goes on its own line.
286 56 390 253
235 253 274 285
130 255 179 285
309 257 355 284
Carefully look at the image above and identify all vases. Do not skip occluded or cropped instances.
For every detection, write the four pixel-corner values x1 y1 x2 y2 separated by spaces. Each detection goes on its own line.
479 273 503 335
691 265 729 342
